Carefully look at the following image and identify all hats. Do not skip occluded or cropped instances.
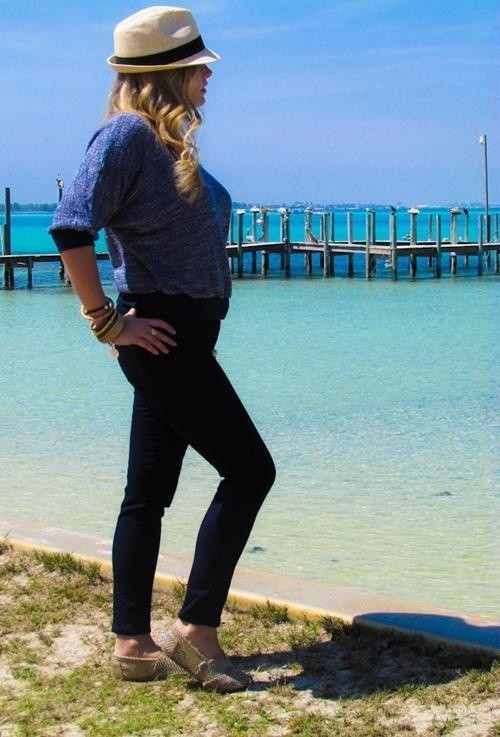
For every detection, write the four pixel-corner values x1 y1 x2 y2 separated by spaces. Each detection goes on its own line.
106 5 220 74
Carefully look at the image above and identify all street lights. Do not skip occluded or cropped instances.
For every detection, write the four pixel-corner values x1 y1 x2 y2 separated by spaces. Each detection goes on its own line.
479 133 488 243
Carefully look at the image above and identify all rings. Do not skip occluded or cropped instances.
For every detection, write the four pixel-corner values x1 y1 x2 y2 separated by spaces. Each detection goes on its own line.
150 328 157 336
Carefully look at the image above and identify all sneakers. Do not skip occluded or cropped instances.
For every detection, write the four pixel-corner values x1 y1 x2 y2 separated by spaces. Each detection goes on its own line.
162 628 248 692
110 652 187 681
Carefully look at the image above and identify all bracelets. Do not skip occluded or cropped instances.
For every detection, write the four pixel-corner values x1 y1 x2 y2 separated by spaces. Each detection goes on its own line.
81 296 126 358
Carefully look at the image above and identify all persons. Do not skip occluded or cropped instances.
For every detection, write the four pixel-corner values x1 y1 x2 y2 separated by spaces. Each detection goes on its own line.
47 5 276 691
